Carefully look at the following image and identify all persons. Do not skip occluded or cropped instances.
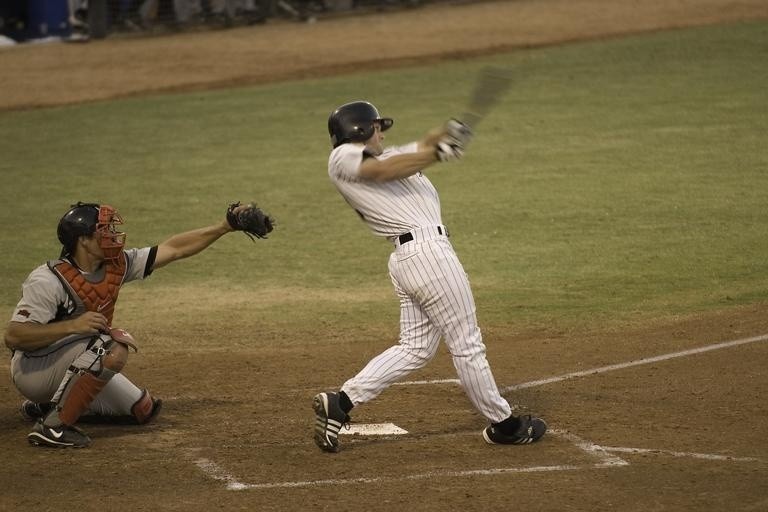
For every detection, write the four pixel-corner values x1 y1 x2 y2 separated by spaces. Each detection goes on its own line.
3 202 281 448
311 101 549 451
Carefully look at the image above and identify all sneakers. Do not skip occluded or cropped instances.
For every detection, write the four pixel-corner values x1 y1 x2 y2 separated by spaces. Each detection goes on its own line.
21 400 49 419
482 416 548 445
27 417 91 450
312 391 352 454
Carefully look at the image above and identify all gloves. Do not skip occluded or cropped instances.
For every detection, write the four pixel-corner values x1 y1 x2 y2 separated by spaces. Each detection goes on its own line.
435 116 474 164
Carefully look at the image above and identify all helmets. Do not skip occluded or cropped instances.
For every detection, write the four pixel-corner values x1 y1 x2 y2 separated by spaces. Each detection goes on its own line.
328 101 393 148
57 201 127 261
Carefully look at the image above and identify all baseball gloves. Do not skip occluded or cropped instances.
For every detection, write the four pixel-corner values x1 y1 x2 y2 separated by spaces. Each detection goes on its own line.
227 201 276 242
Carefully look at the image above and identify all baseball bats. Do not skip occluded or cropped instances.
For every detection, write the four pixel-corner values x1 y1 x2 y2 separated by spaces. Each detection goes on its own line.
463 67 512 131
